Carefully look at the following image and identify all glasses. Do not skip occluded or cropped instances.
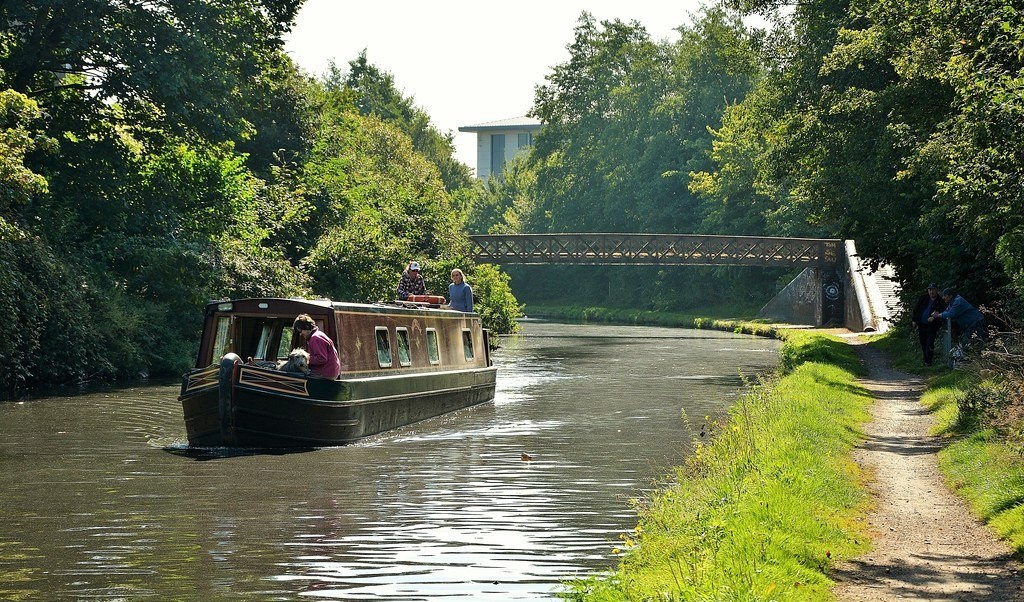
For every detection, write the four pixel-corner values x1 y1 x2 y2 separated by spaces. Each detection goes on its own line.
296 328 302 333
452 274 460 276
410 270 418 272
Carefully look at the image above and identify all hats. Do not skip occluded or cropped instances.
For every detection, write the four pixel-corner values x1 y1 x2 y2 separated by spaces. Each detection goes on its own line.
409 262 420 271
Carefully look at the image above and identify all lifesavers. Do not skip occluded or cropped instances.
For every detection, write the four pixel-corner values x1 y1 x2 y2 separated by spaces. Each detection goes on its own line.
408 294 446 305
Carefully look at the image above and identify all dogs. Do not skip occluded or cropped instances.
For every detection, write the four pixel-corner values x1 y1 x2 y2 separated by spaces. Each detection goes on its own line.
278 347 310 373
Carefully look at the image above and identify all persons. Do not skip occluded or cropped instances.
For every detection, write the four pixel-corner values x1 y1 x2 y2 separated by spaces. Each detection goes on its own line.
912 283 986 366
448 269 473 313
396 262 430 301
294 314 341 380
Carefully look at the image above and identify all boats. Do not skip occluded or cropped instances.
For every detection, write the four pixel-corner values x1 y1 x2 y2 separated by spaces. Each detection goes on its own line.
177 295 498 447
